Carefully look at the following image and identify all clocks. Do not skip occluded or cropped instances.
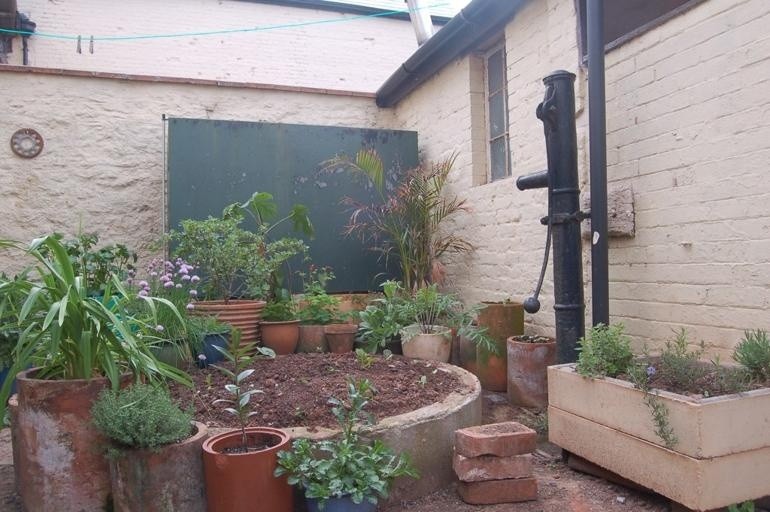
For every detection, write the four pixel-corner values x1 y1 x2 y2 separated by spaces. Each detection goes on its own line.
9 128 45 158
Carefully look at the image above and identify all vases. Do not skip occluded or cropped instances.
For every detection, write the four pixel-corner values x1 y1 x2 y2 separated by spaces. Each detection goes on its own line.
146 335 188 374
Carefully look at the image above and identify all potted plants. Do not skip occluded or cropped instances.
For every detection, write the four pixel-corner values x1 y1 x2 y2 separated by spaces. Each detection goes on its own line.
0 228 147 510
260 261 299 349
477 290 524 395
505 332 556 408
394 149 454 362
292 372 405 512
91 371 206 503
297 259 339 352
167 207 269 354
199 330 299 510
189 317 232 366
322 285 356 355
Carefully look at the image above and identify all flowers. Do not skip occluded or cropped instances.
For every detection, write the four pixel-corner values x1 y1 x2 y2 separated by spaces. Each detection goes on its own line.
128 255 198 333
